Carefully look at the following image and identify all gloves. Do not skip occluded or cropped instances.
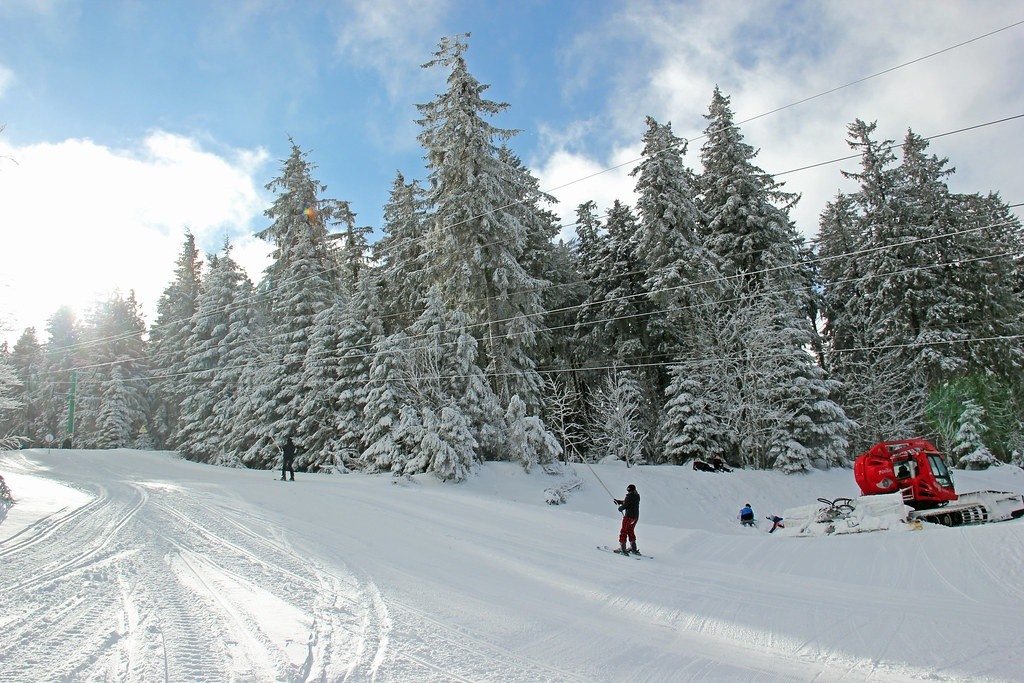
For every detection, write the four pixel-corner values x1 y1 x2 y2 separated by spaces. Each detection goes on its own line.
613 498 622 505
618 505 625 511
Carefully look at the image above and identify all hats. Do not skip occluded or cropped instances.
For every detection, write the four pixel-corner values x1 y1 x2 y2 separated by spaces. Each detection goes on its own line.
287 438 293 442
745 503 751 507
626 484 637 491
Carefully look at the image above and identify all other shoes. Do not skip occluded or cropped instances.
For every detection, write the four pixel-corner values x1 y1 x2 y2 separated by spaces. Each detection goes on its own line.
289 477 294 481
280 476 286 481
625 543 637 553
614 544 626 553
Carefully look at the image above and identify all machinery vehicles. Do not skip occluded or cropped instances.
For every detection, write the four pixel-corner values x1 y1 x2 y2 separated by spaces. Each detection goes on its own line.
854 439 987 527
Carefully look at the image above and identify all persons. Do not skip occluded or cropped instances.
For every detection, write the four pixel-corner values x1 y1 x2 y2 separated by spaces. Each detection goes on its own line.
613 484 640 556
280 438 295 481
766 514 784 533
741 504 754 526
